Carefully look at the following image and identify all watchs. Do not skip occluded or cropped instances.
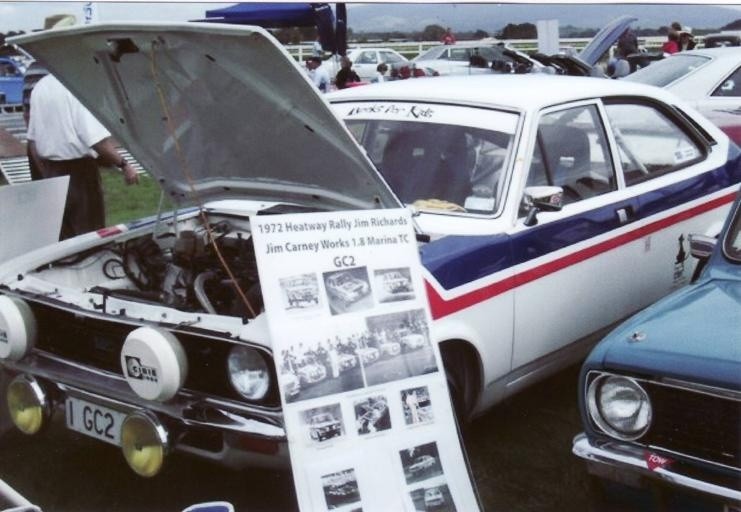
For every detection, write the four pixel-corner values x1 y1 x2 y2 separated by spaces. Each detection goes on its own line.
117 158 128 168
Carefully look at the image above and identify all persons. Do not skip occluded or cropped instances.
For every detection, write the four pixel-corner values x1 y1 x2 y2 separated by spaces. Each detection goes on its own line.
614 23 695 76
25 74 139 241
282 328 386 373
405 390 421 424
301 49 528 92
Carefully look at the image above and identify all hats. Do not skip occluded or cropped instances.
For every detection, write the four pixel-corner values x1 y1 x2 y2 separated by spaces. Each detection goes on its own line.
675 26 694 38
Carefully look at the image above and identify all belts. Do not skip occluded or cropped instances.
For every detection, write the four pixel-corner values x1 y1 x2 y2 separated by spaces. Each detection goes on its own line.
39 156 94 173
